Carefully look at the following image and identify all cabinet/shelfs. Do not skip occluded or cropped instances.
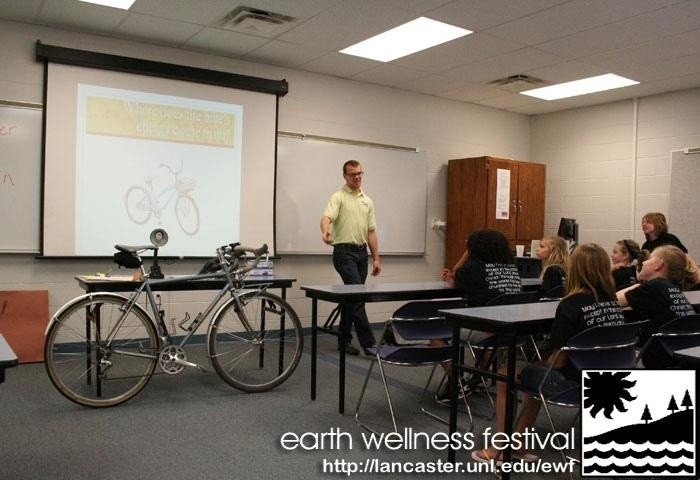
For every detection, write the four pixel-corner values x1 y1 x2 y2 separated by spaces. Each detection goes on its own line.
444 153 546 281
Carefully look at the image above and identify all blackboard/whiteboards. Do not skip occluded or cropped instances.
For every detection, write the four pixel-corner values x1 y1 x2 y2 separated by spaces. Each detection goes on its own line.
0 99 428 257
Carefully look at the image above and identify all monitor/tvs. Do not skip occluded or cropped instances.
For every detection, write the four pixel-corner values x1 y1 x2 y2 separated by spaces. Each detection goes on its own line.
557 217 578 240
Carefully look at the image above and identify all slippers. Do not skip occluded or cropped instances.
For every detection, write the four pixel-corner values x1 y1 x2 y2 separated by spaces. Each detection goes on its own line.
470 446 538 467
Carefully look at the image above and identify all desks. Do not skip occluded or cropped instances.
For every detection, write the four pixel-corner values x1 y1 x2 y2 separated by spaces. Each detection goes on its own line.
70 266 302 400
0 332 20 385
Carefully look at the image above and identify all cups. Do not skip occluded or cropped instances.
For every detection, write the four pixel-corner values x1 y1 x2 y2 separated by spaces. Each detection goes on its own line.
516 245 524 256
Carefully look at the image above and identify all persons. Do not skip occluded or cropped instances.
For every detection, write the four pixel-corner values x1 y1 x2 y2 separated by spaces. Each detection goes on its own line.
320 160 382 358
428 212 699 468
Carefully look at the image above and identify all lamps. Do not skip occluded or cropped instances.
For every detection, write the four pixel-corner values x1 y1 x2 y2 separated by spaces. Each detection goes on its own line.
146 226 174 279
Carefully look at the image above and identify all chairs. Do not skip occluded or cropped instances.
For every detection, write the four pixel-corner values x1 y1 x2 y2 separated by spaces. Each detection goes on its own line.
296 271 699 480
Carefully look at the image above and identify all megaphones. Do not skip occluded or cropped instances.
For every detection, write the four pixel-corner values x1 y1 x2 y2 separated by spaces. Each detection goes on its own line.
150 229 169 248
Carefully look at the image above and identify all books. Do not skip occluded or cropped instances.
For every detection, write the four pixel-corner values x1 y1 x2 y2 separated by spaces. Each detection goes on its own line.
248 259 274 275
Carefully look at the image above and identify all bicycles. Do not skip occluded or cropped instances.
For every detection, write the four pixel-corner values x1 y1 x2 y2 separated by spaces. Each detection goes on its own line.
43 242 304 408
124 158 200 236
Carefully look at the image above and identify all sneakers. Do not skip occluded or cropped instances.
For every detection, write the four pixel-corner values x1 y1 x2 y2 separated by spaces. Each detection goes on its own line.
434 378 490 403
337 341 360 355
